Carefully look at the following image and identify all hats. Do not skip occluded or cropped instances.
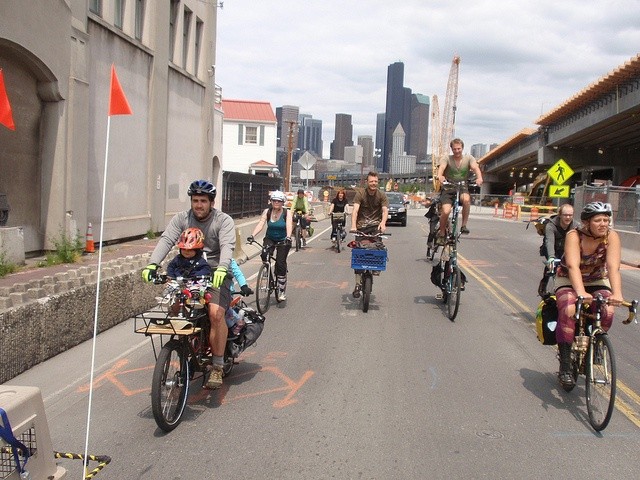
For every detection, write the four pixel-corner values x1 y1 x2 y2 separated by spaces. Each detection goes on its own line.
297 189 304 194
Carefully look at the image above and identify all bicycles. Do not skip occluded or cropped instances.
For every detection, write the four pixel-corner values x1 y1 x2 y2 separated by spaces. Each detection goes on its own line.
349 230 391 313
436 178 478 321
426 219 453 261
246 238 291 315
329 212 349 253
131 271 254 431
293 213 306 252
555 293 638 431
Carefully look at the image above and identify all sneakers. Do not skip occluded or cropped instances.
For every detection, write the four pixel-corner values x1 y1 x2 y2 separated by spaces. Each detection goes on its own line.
206 367 223 388
440 237 446 246
459 227 470 232
352 285 361 298
373 270 381 277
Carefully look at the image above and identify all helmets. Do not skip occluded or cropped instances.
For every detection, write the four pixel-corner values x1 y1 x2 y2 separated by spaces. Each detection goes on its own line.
269 191 287 203
177 228 205 249
187 181 216 201
580 201 611 219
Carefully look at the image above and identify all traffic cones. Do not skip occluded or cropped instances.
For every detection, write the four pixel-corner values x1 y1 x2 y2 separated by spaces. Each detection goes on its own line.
84 222 96 253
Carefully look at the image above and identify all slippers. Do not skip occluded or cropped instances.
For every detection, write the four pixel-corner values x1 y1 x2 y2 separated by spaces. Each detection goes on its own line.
557 341 578 391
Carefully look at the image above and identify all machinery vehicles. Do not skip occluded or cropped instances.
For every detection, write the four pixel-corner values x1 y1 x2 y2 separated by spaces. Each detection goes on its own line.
515 170 552 211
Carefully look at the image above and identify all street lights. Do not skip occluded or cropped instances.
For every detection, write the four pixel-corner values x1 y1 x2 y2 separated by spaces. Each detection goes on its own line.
372 148 382 173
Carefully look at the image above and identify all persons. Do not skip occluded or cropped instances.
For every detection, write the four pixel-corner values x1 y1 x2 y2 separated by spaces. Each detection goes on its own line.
328 190 350 247
539 204 583 297
290 189 308 246
425 188 457 258
141 180 236 388
231 258 252 296
247 191 292 301
437 139 483 246
555 202 624 388
350 172 388 298
167 227 211 277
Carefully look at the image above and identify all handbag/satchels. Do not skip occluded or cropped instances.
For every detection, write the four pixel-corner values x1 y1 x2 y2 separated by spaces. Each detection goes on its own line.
430 263 443 285
229 307 264 358
536 296 558 344
304 228 313 237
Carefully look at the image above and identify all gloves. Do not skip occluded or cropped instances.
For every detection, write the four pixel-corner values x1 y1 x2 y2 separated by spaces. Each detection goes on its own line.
142 265 158 283
210 267 226 287
243 286 252 295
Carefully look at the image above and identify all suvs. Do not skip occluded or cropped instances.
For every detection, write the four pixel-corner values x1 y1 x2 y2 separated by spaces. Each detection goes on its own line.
384 192 409 226
